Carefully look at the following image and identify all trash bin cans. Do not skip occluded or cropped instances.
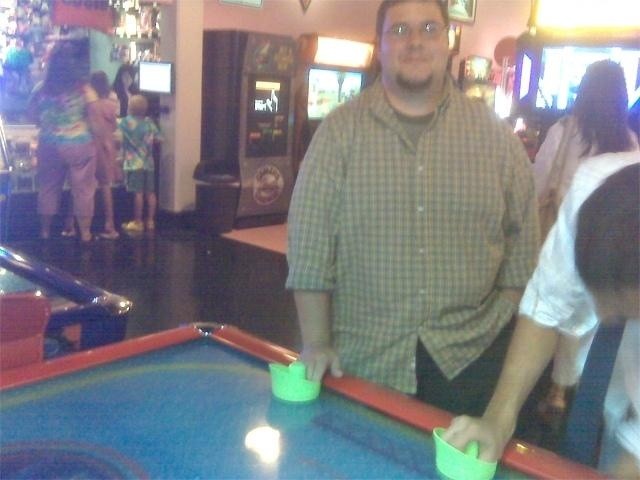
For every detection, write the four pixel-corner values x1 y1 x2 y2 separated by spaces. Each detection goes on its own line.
193 159 240 233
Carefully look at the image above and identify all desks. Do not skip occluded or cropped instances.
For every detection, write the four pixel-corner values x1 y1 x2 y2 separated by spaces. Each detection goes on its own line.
0 322 624 480
0 247 133 360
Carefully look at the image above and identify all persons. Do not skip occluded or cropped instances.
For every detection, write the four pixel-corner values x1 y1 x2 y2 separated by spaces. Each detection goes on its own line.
283 0 542 436
534 59 640 249
117 94 164 232
445 149 640 480
60 68 123 239
25 40 98 243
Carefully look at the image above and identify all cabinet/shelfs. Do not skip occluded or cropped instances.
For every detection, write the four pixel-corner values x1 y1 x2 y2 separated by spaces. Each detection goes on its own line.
0 120 126 244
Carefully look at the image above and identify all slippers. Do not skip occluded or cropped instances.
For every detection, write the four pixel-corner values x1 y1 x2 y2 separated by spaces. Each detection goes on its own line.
35 229 119 244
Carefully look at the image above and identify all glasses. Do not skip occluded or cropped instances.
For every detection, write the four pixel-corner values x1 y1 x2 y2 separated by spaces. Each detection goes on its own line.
377 21 450 39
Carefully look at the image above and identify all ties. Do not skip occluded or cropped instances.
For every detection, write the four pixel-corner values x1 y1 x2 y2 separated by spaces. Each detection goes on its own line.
561 320 628 463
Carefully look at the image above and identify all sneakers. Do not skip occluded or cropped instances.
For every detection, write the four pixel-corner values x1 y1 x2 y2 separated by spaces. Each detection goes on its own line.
121 219 156 233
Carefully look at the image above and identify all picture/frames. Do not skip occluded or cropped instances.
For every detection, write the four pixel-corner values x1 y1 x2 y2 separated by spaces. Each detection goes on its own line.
447 0 476 24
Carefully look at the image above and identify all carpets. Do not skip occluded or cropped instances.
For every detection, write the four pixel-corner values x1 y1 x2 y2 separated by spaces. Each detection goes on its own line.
219 222 288 257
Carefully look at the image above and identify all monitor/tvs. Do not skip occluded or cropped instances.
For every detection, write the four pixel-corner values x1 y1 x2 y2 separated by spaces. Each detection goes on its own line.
535 45 640 115
513 47 534 102
308 68 362 121
254 80 280 112
138 61 173 96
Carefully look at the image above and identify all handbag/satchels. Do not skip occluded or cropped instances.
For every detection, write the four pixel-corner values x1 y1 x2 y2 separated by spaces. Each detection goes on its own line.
534 113 577 245
